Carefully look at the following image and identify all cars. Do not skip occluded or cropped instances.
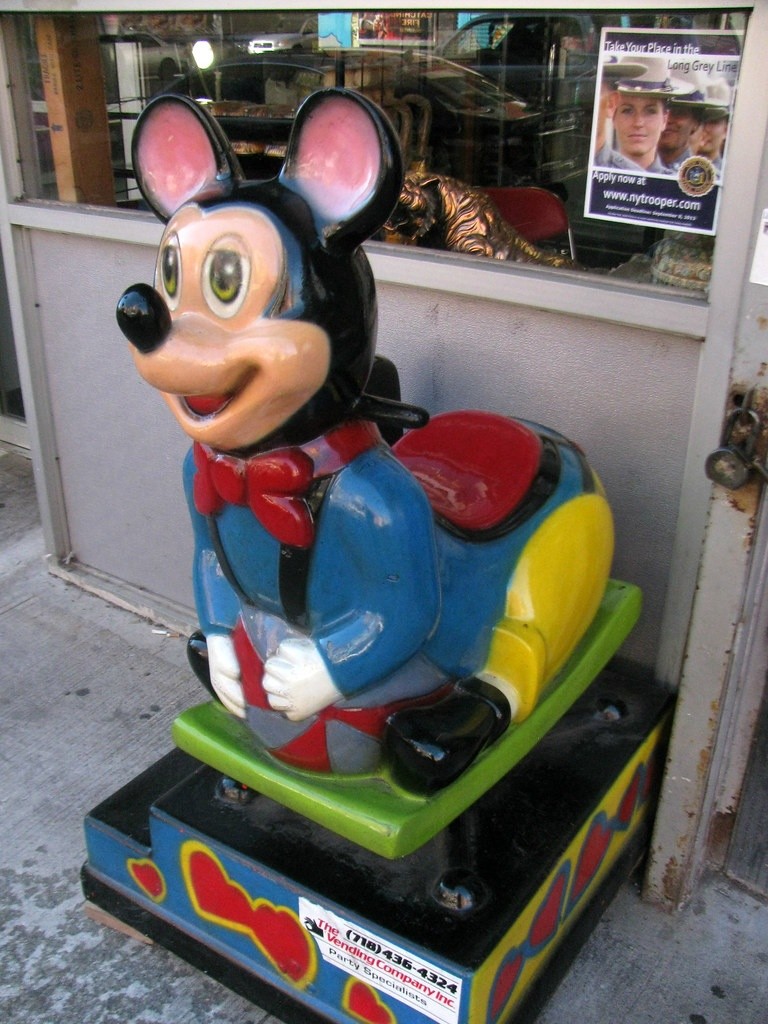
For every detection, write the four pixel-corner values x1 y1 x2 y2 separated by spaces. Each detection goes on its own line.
101 9 678 197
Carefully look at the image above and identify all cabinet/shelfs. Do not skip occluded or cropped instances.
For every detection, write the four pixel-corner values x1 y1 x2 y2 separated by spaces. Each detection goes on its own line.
99 29 346 181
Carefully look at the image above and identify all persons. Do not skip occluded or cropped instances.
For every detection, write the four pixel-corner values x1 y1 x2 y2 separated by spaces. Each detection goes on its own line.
361 13 387 39
595 55 731 181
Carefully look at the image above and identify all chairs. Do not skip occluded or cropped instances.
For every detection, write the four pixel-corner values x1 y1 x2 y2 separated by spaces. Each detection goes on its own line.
475 187 575 259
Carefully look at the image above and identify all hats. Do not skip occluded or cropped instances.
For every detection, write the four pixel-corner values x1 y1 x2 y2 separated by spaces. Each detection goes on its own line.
603 51 732 121
